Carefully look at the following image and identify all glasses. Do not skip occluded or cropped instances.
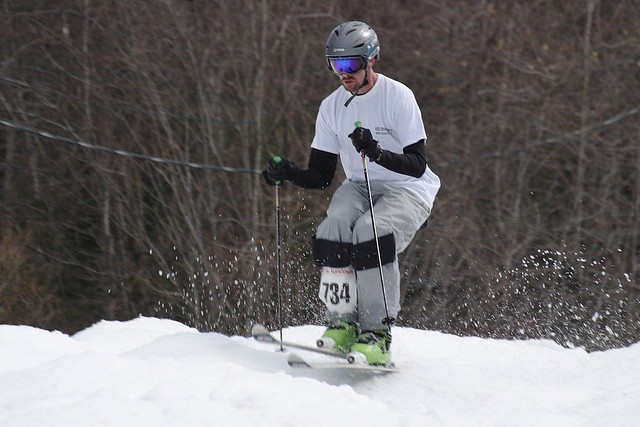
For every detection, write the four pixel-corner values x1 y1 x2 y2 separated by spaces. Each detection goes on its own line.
328 57 366 77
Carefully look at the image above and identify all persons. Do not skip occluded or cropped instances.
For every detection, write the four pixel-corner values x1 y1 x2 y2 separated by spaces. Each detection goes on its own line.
262 20 441 368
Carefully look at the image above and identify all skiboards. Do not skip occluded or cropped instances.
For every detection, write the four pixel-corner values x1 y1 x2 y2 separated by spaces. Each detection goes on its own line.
251 323 399 372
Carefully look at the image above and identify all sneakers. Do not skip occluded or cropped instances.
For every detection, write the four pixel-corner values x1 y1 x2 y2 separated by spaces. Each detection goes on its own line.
349 328 392 365
322 319 359 355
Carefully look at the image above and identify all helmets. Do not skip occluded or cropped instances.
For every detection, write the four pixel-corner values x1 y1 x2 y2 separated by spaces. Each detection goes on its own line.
325 21 381 72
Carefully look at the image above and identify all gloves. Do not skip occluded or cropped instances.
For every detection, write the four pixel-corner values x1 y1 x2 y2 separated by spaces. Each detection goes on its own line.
266 157 298 185
348 127 381 162
